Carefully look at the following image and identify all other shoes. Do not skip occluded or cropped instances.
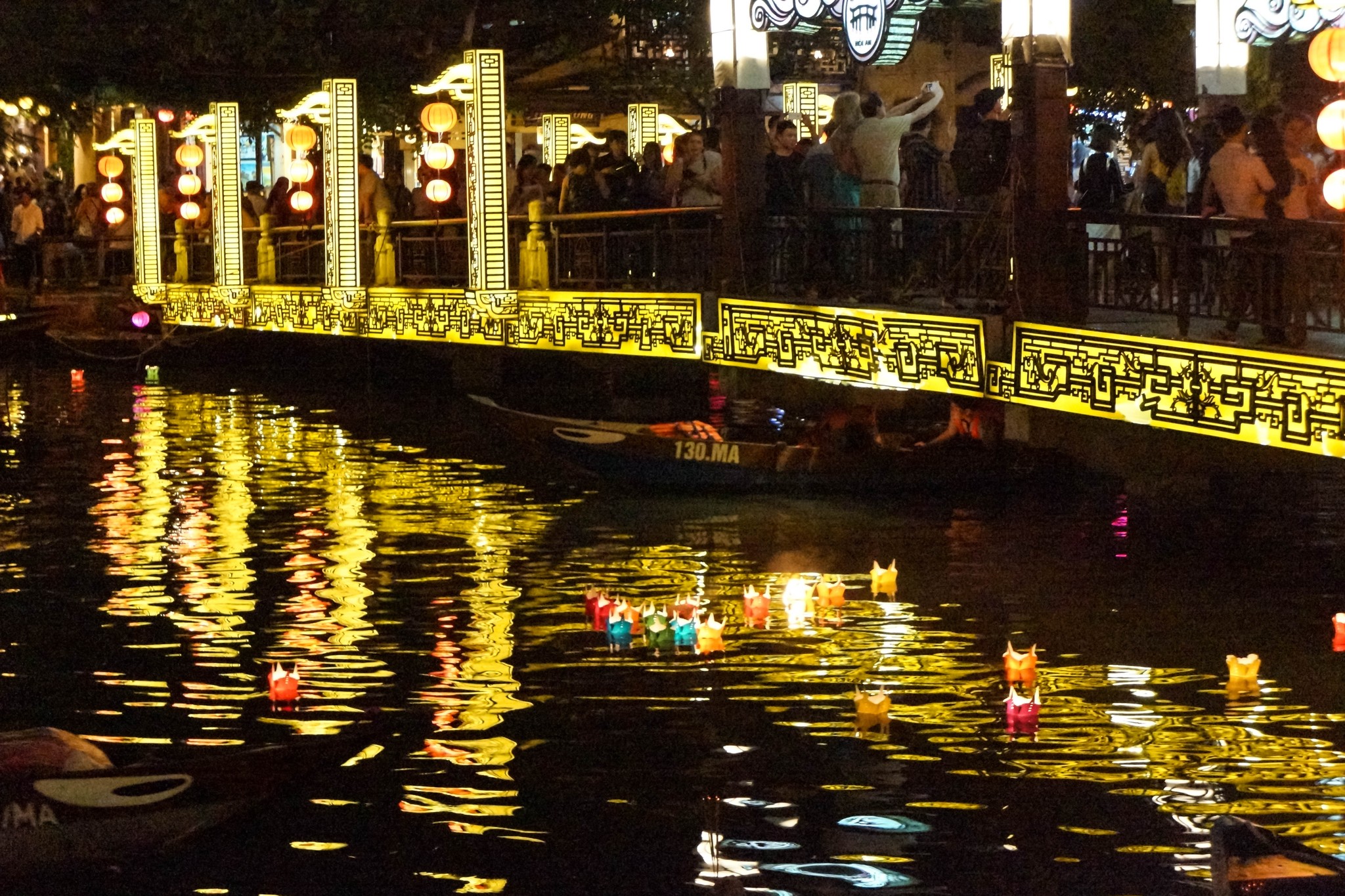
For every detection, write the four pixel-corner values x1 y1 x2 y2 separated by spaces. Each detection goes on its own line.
1257 331 1288 345
942 295 965 310
1212 329 1235 341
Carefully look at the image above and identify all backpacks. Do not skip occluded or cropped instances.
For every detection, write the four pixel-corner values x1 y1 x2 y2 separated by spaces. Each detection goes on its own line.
568 168 607 229
950 120 1006 195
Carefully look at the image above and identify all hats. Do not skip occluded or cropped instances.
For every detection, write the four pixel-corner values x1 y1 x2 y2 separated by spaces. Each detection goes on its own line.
974 87 1005 112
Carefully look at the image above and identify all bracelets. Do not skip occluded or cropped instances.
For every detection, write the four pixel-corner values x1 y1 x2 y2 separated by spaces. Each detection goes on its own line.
915 96 918 103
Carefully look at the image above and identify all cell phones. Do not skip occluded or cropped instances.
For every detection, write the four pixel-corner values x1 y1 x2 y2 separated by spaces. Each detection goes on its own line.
928 84 932 91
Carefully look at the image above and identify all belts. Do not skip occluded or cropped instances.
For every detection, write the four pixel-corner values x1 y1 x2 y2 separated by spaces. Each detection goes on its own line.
862 179 896 186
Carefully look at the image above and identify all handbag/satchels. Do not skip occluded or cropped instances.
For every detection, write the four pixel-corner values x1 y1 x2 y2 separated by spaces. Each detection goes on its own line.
1140 171 1167 213
92 210 108 240
1077 184 1114 209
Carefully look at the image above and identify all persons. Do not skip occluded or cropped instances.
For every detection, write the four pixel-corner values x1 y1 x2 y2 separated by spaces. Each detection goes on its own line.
0 79 1332 348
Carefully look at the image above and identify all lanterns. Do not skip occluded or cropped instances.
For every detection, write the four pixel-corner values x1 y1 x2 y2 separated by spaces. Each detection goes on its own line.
175 143 204 168
424 142 455 170
288 160 314 183
101 182 123 203
426 179 451 201
98 155 124 177
291 191 313 210
1317 99 1345 151
106 207 124 224
178 174 201 195
1322 168 1345 210
421 103 457 132
285 125 316 151
1307 27 1345 82
180 202 200 220
